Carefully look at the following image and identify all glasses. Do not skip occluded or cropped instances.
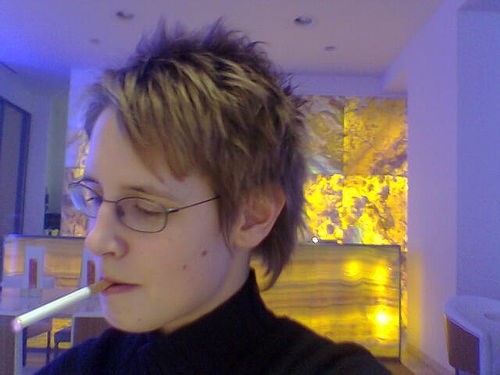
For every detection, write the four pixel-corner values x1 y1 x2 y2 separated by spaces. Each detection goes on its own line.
68 172 222 234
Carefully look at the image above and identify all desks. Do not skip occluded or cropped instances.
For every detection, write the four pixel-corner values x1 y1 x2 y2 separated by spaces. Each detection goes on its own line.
1 286 109 375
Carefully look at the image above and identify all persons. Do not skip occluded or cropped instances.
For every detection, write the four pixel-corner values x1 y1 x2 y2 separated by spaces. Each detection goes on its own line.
30 12 392 375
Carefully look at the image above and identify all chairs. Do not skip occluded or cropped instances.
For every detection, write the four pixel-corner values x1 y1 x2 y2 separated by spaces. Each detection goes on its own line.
1 275 56 367
54 326 72 359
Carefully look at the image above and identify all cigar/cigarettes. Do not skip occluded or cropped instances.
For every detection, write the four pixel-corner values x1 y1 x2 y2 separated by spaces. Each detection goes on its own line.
12 279 114 331
